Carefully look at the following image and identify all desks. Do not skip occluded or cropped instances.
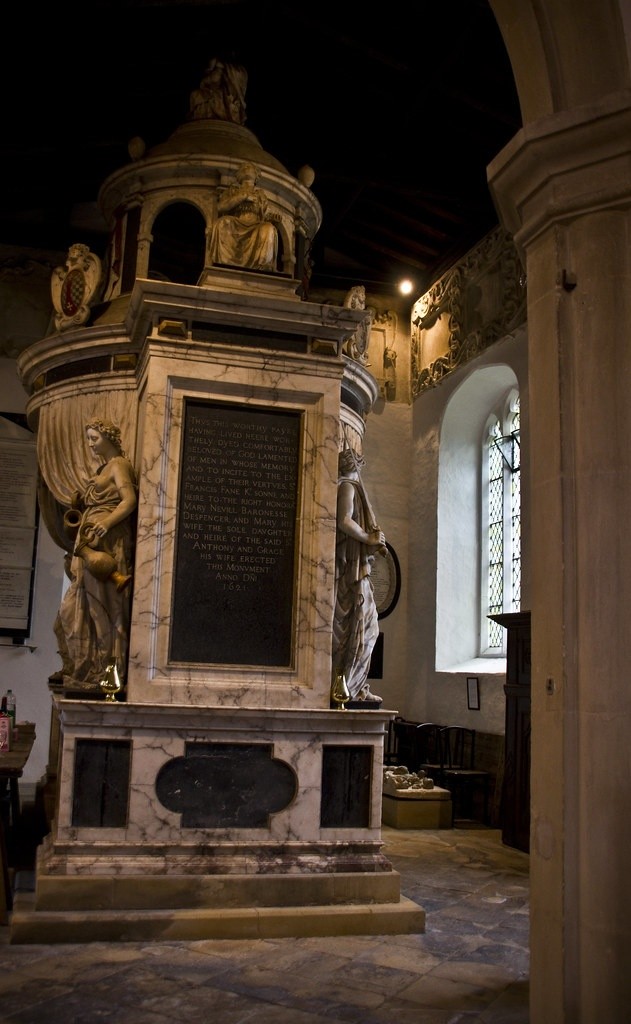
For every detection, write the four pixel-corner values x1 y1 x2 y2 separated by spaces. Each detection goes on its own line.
382 765 453 829
0 722 38 926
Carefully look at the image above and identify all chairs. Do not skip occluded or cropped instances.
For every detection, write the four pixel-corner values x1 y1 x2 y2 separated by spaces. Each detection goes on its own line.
416 721 461 786
384 716 411 767
435 726 491 827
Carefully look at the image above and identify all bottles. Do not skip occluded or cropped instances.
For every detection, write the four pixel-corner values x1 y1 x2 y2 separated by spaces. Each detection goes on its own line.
3 689 16 729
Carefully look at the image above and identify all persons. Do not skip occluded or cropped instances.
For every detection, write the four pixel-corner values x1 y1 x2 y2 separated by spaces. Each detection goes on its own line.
209 158 282 271
332 446 389 702
295 247 314 300
47 415 137 686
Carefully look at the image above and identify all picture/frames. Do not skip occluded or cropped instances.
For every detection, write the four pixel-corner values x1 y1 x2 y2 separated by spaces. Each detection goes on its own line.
467 678 481 710
